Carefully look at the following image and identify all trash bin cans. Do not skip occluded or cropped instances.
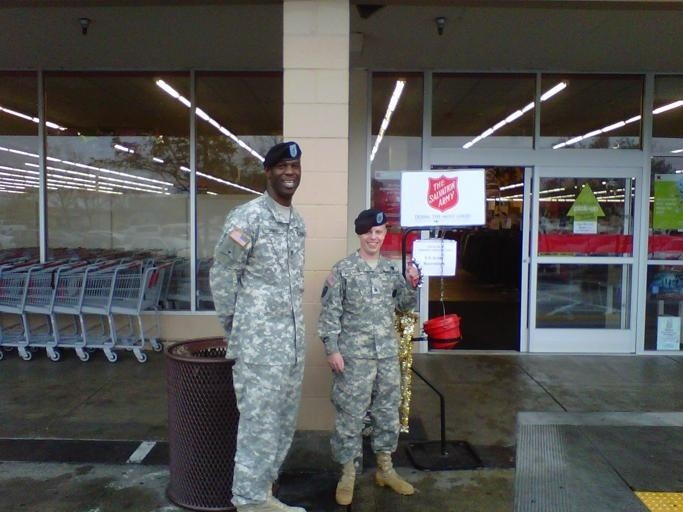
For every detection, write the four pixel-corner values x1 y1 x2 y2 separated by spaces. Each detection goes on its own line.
163 336 279 511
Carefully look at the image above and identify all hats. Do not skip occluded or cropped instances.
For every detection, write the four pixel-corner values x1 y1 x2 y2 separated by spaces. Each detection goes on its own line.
263 142 301 168
356 209 388 235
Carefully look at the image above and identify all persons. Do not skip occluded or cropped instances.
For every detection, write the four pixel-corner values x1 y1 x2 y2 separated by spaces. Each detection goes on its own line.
316 207 422 510
207 138 310 512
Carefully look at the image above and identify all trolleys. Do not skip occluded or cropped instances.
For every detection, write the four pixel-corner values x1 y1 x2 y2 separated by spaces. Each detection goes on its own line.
0 250 174 365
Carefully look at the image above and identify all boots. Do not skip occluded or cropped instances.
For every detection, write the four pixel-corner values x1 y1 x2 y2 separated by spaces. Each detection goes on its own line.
336 459 355 505
237 484 307 512
374 451 414 495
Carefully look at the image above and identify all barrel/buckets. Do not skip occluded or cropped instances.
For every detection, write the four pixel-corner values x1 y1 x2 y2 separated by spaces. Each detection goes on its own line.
423 313 462 349
